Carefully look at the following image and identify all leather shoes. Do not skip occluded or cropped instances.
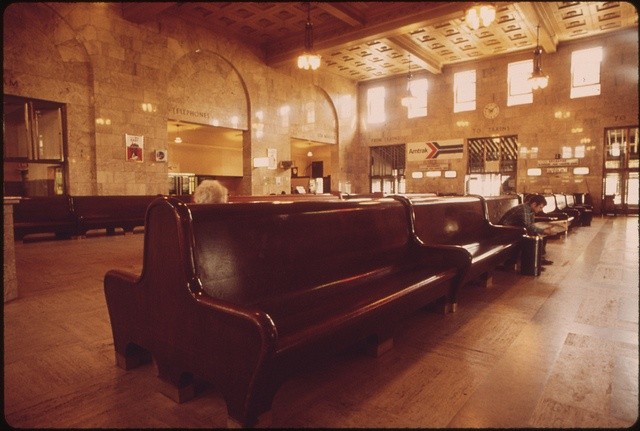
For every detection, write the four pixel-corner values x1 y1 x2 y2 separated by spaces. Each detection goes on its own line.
540 266 546 271
541 260 553 265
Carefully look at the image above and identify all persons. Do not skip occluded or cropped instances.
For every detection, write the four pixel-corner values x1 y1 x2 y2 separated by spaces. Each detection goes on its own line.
193 179 228 204
498 194 554 270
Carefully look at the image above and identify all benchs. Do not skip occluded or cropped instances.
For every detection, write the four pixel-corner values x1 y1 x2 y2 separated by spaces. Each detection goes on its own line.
105 192 550 424
522 192 595 234
12 188 166 243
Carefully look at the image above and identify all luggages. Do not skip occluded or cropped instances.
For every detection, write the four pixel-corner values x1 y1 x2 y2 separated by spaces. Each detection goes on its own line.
520 230 543 277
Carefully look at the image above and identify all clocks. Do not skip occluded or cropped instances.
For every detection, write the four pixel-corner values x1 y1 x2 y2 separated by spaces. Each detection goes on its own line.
483 102 501 120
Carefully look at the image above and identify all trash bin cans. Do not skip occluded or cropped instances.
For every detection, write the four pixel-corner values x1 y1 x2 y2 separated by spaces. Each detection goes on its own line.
581 210 592 228
522 234 543 277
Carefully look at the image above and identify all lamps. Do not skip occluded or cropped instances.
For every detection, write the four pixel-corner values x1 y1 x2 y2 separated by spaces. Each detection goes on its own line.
527 23 550 91
460 1 496 31
296 3 321 72
